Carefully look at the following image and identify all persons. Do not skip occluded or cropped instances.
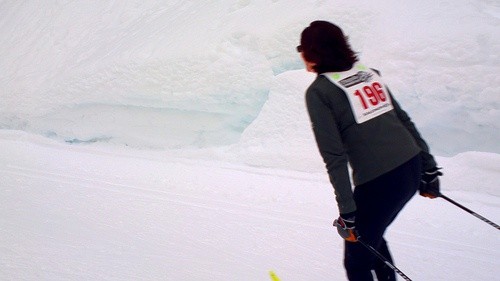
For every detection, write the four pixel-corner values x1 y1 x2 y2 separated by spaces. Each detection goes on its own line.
297 19 443 281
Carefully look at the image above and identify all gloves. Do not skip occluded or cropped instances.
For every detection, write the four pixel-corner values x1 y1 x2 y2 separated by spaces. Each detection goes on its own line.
336 212 360 242
418 167 443 198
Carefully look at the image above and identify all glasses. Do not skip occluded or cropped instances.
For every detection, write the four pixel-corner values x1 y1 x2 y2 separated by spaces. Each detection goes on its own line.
297 45 302 52
